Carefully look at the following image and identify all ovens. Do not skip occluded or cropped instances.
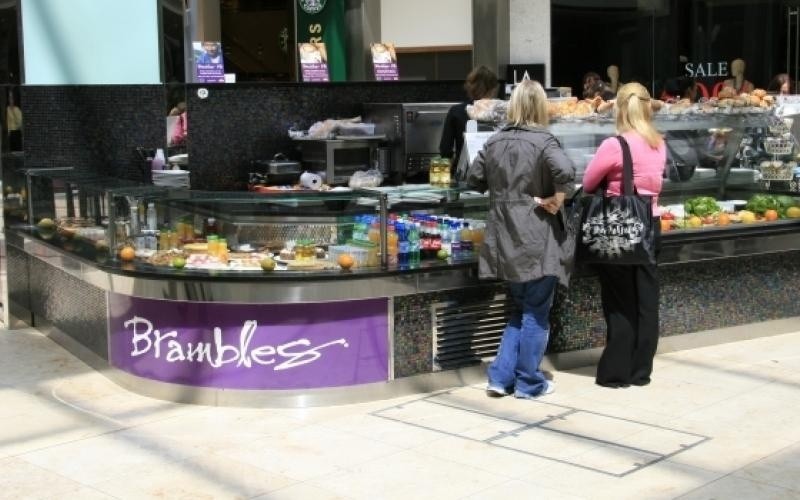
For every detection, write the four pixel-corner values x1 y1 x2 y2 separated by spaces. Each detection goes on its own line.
364 102 465 175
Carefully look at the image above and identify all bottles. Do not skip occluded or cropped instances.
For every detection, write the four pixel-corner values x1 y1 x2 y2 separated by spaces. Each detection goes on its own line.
353 211 486 268
440 159 451 188
295 245 314 264
661 213 674 231
146 203 158 231
161 221 196 249
129 206 141 236
430 158 441 185
206 217 227 257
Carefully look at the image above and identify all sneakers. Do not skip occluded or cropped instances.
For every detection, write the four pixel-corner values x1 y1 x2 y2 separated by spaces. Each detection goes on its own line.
628 370 650 385
514 380 555 398
486 377 515 398
593 373 629 388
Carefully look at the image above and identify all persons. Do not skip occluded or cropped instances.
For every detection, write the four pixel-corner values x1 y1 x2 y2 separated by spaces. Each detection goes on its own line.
439 66 500 177
196 41 222 64
604 64 626 99
583 81 667 388
721 59 754 96
465 81 576 399
665 76 698 103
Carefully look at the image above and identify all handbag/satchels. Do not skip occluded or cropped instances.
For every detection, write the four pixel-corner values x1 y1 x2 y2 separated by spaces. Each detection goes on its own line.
578 134 651 265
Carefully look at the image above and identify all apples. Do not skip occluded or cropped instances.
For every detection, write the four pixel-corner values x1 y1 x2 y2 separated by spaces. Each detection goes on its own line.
437 250 448 259
173 258 185 269
261 259 277 271
95 240 107 252
38 218 54 229
660 205 800 231
121 247 134 261
337 255 354 269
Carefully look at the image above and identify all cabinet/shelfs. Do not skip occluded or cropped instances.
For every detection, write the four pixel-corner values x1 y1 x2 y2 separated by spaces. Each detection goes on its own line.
1 165 490 278
546 102 800 278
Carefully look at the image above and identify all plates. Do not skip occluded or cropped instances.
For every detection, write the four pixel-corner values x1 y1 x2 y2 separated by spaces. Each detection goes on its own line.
273 252 330 265
728 199 747 205
183 243 274 260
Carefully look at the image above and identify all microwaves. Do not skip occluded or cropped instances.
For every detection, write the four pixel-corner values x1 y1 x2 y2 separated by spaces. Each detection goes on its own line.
302 142 378 183
